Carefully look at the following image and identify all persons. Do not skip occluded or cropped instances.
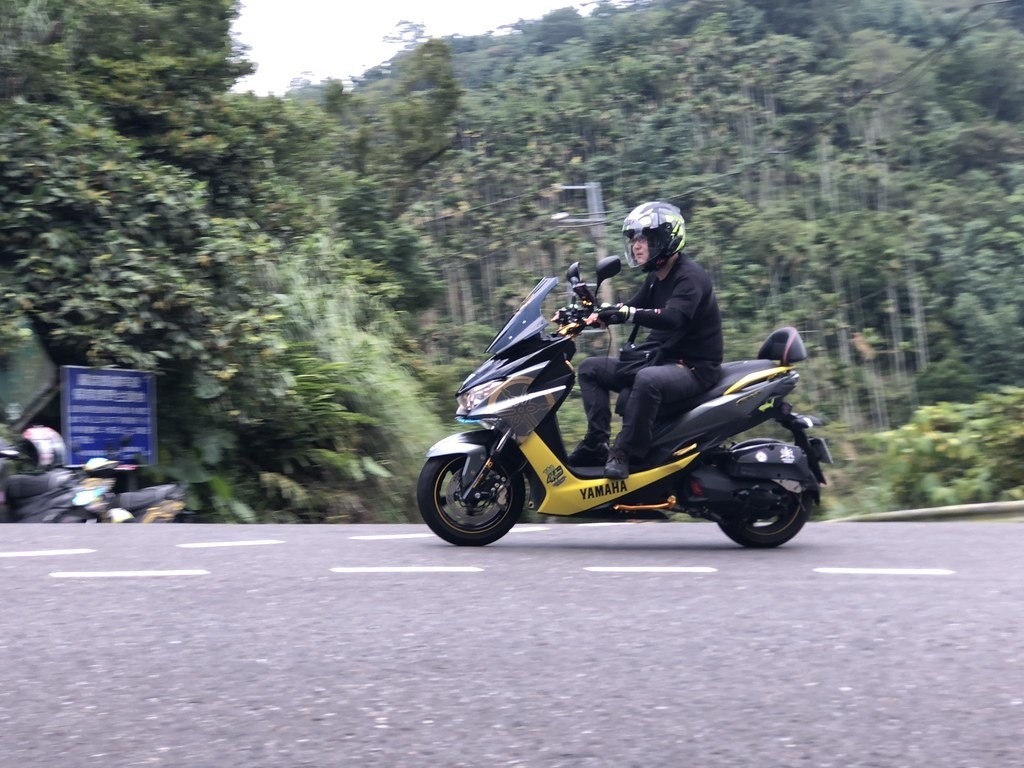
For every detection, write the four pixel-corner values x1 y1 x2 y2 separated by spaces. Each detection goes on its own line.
551 201 723 479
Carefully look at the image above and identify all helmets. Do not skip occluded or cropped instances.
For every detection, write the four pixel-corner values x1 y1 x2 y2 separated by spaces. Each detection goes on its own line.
17 425 68 474
622 202 686 272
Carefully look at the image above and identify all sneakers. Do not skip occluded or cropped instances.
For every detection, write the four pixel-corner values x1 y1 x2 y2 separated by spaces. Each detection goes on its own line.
567 439 610 467
603 447 630 479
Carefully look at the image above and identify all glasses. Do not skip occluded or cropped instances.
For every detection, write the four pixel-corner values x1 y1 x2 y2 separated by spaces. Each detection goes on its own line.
628 235 647 243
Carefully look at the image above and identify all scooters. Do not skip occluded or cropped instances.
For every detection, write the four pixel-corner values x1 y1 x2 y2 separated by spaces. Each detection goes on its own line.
70 428 193 523
2 446 124 523
415 255 835 548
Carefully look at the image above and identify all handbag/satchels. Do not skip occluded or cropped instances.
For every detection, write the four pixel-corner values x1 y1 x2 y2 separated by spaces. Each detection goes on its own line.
614 341 666 379
